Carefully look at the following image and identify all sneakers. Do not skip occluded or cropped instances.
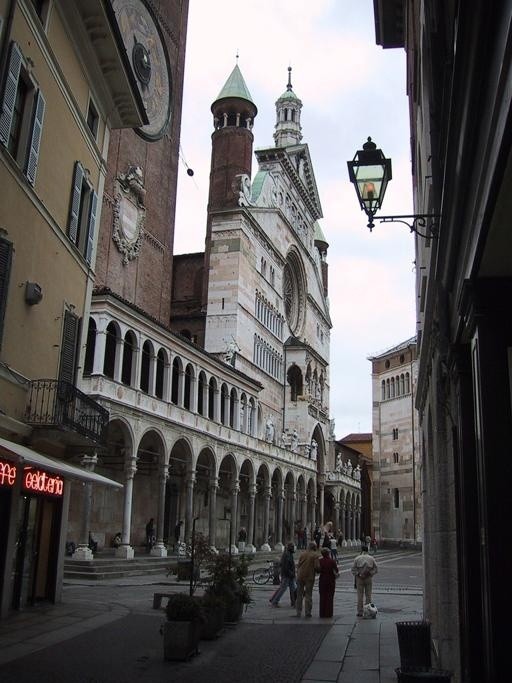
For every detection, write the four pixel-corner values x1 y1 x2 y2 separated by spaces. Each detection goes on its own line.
357 614 363 617
267 599 280 609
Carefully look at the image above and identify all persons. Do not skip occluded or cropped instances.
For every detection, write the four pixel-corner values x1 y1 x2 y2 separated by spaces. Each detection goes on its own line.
365 533 372 550
238 526 247 541
336 451 362 480
145 517 154 552
372 538 378 551
351 546 379 616
360 531 366 546
266 520 345 564
112 531 122 544
88 529 98 551
292 540 319 617
270 541 297 606
320 547 339 617
173 520 184 550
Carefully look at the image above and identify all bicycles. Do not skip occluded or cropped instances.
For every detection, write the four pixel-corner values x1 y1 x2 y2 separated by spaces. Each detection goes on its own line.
252 557 282 585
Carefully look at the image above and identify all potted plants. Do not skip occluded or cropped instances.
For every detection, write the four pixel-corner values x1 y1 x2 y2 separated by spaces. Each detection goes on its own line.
208 572 245 626
194 587 227 641
161 591 202 660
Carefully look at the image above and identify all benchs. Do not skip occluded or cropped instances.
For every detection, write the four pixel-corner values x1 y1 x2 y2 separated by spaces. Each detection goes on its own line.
154 592 198 609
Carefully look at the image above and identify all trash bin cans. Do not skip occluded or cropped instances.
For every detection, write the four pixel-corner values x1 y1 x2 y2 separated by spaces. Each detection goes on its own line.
394 621 454 683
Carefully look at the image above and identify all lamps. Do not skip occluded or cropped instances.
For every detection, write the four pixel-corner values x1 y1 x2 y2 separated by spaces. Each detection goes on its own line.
344 133 441 242
